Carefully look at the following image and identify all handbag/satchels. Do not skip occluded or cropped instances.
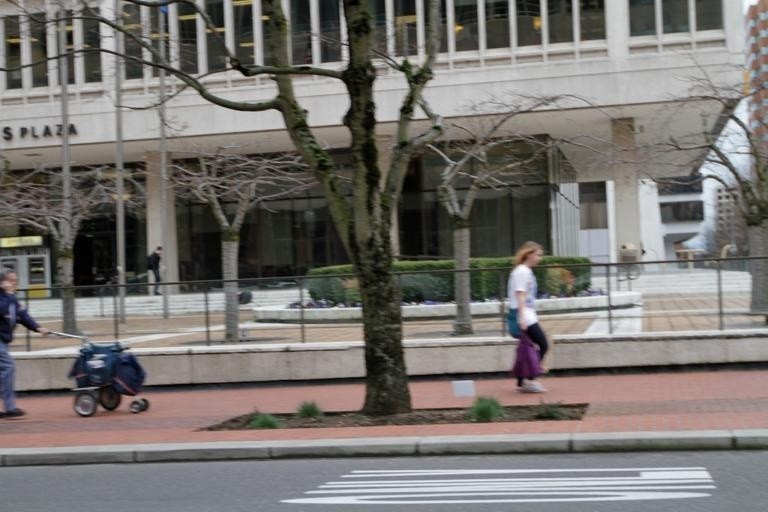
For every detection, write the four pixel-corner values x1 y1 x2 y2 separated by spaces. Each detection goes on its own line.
506 308 522 338
511 346 547 377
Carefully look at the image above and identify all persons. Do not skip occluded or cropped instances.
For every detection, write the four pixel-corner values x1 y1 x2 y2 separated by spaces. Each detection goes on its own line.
502 240 550 395
87 265 108 293
145 246 163 296
0 266 53 419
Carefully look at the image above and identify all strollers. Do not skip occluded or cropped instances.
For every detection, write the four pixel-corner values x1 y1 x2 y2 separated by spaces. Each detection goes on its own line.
65 334 150 418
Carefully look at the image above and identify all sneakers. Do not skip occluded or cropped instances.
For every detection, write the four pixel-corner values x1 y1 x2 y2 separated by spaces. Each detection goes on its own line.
515 380 546 396
0 409 25 419
